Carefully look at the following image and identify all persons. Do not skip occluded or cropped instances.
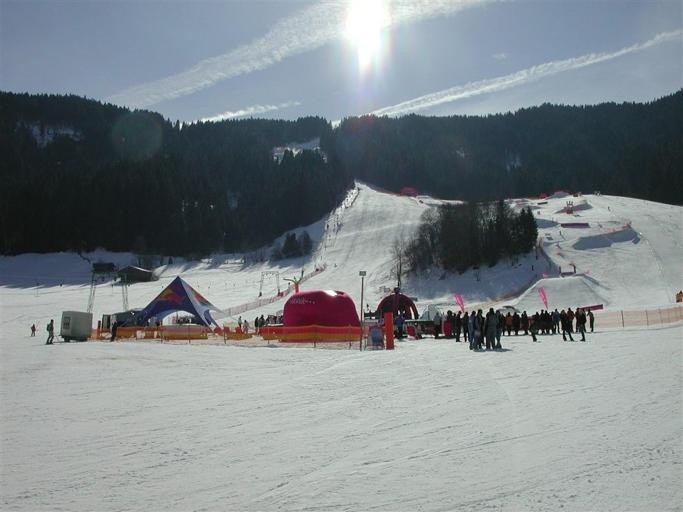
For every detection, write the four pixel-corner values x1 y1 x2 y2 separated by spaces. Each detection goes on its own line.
109 322 117 342
431 307 593 351
394 314 402 339
568 262 576 273
45 319 54 345
30 324 36 337
237 314 282 334
557 264 561 274
